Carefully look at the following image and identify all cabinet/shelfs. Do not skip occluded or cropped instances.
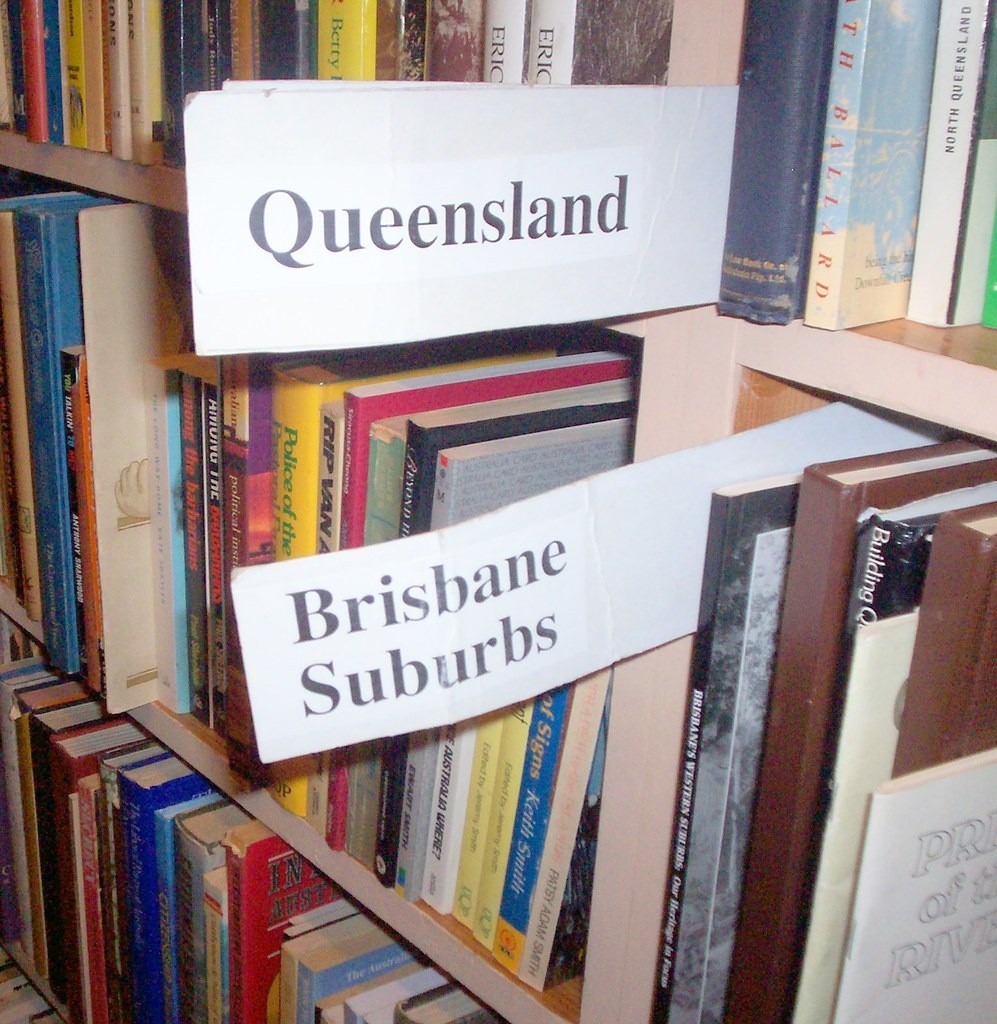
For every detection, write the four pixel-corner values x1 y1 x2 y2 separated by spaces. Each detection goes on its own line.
0 0 997 1024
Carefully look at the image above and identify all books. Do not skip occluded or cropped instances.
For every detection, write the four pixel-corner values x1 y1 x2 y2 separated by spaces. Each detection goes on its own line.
647 471 801 1024
0 0 997 1024
